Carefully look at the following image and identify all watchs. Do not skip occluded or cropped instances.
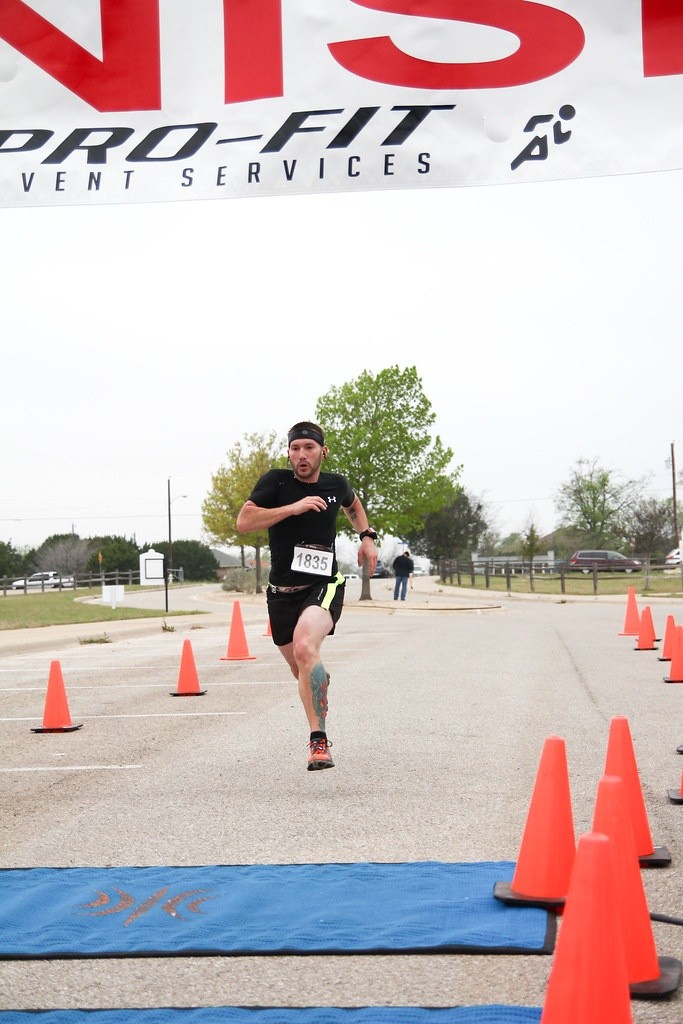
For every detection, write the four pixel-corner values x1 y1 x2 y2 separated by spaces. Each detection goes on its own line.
360 528 377 540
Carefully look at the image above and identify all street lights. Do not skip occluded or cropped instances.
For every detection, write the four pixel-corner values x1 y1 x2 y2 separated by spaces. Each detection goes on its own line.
167 478 187 567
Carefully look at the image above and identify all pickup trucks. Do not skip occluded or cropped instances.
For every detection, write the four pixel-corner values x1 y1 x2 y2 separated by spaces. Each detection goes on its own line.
11 571 74 590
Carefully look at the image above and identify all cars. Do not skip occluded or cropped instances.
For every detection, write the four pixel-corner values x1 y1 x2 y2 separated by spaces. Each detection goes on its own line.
369 558 394 579
342 574 359 580
568 549 643 575
412 565 428 577
664 547 683 570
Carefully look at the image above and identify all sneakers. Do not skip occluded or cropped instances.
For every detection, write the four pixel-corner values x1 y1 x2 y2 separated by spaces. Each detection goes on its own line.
305 738 335 771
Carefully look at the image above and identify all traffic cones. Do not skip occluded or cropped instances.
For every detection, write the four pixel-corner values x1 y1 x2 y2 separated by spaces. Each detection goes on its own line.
492 734 577 908
219 600 257 661
29 659 84 733
538 832 634 1024
633 606 662 651
262 614 273 636
592 774 682 1000
169 638 208 696
601 716 673 869
656 615 676 661
617 586 641 636
662 626 683 684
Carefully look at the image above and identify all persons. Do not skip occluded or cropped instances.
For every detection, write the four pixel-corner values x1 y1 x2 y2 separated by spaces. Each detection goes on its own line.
237 423 377 769
393 552 414 600
